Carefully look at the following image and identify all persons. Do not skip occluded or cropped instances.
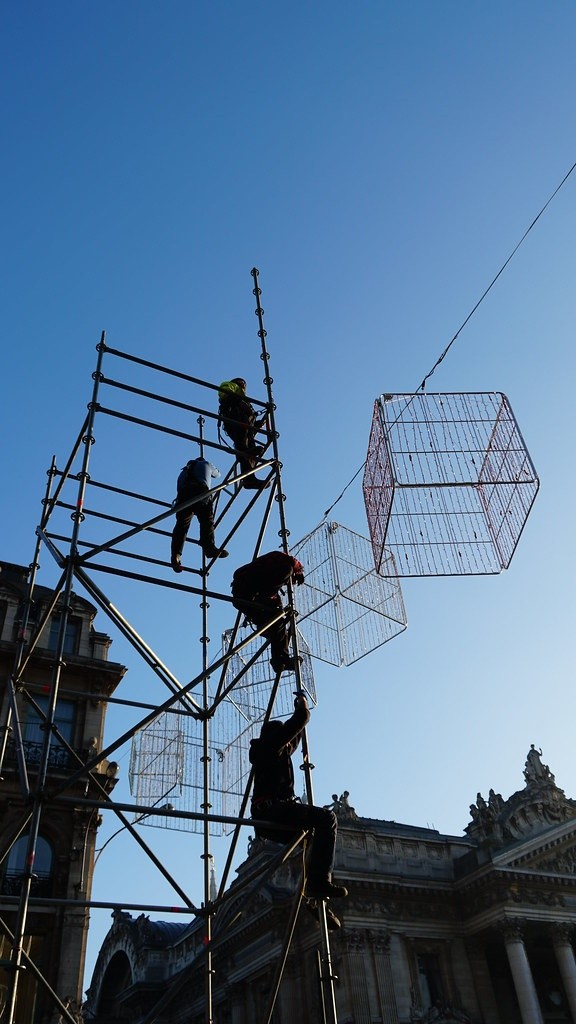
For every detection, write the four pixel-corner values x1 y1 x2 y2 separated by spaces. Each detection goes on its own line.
229 551 304 672
218 377 273 490
250 688 348 932
170 456 231 573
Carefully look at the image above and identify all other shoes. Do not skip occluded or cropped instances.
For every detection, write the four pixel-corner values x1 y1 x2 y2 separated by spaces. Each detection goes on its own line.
171 554 182 573
255 595 275 608
243 477 270 490
302 898 341 930
304 872 348 898
271 655 303 674
249 445 263 456
205 545 229 559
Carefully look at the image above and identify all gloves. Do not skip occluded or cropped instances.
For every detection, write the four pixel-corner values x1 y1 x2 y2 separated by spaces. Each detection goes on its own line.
293 689 307 711
293 573 305 587
